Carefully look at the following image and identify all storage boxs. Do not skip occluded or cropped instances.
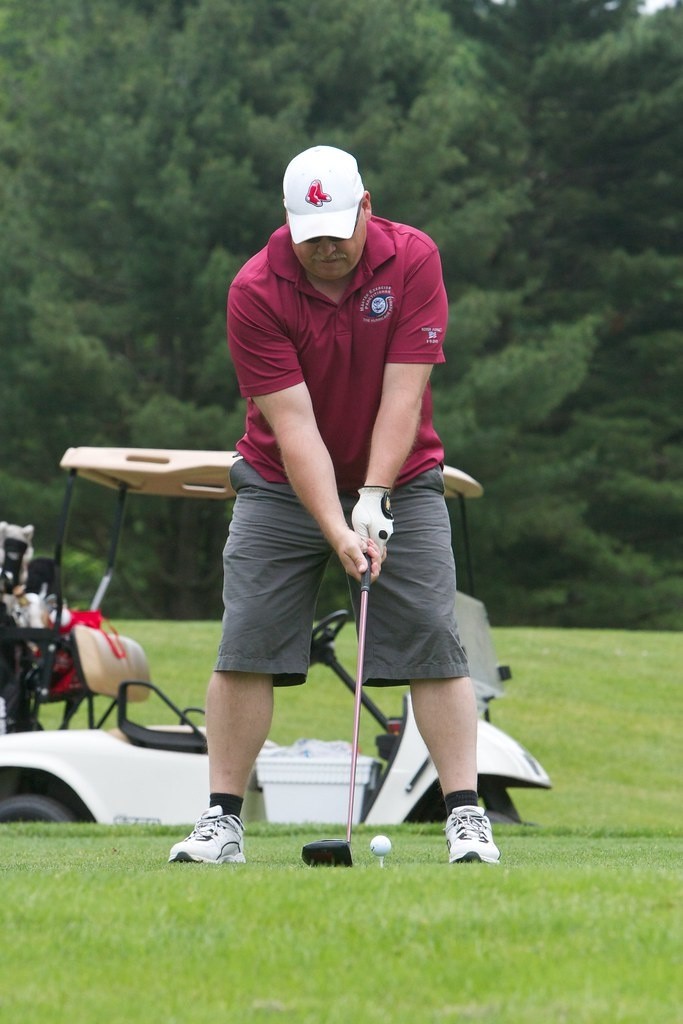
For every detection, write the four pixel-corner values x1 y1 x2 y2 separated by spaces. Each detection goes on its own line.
252 753 375 826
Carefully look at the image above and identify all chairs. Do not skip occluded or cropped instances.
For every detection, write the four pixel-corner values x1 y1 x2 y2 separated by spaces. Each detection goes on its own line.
69 621 210 752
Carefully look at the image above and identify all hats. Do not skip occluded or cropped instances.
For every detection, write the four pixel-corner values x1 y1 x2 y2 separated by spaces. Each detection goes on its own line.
282 145 364 244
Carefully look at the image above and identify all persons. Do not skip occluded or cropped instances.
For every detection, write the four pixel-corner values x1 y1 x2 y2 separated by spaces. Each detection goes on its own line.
167 144 503 865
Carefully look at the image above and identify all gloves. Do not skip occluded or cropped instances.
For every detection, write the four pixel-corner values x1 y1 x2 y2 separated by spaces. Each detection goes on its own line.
351 485 395 556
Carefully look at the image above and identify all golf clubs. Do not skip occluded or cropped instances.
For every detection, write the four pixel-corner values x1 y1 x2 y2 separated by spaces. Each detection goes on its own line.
300 553 373 868
4 582 69 629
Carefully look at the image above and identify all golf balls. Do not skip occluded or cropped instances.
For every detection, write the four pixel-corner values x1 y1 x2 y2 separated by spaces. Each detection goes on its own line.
370 835 392 856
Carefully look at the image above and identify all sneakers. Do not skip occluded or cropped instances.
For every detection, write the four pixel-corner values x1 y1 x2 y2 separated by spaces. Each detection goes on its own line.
168 805 246 864
443 805 501 864
